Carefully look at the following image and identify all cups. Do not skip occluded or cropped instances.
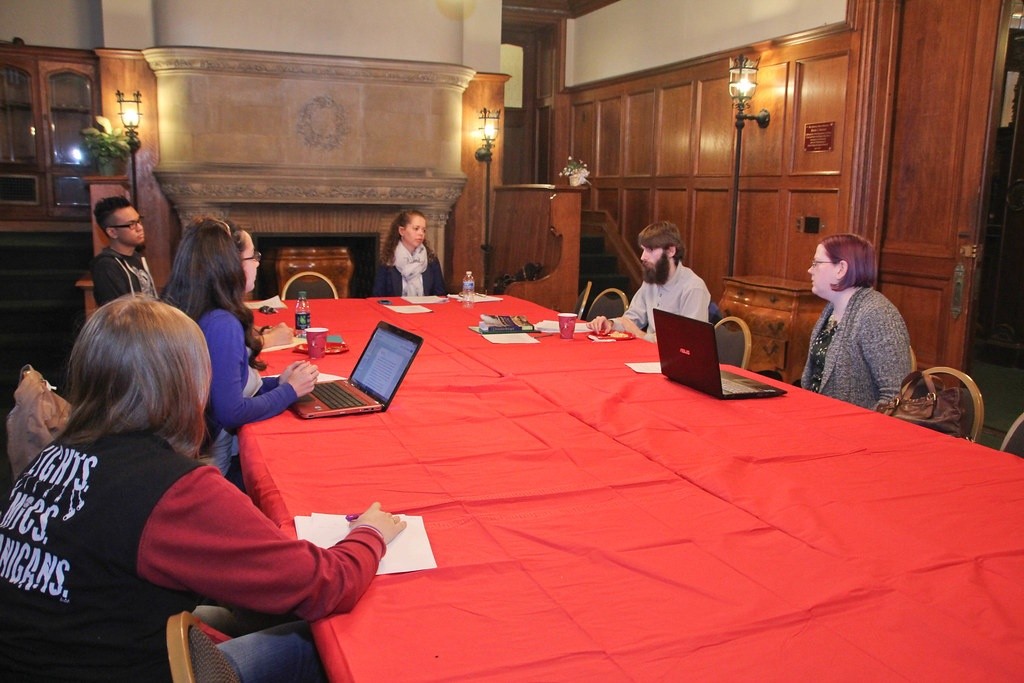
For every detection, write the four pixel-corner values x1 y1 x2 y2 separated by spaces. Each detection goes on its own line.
304 328 329 358
557 313 577 339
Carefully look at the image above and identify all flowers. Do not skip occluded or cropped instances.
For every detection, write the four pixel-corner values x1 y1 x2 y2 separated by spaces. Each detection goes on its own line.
559 156 589 178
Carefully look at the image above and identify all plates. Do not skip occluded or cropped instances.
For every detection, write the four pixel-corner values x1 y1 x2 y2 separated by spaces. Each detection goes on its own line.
295 343 348 355
587 331 635 340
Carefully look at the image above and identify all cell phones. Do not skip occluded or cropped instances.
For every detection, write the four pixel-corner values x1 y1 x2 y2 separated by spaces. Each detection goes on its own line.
379 299 393 305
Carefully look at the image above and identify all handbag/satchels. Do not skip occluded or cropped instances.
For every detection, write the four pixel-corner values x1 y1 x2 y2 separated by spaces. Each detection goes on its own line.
873 370 965 438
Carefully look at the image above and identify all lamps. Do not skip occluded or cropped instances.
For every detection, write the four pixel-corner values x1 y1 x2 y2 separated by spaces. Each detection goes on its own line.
114 89 143 215
479 107 501 281
728 53 770 282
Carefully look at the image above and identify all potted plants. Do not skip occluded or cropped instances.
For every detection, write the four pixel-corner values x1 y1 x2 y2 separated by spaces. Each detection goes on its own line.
81 115 131 177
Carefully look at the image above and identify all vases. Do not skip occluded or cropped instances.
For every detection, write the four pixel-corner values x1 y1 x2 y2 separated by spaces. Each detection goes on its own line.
569 174 581 186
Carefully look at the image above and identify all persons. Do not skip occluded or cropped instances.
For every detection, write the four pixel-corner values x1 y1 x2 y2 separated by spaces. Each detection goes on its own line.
92 195 160 308
156 213 318 496
0 298 407 683
798 232 912 411
371 210 448 298
584 218 712 343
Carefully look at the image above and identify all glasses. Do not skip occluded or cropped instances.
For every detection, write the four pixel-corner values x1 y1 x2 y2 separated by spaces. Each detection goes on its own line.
811 258 839 269
104 215 145 230
240 251 263 262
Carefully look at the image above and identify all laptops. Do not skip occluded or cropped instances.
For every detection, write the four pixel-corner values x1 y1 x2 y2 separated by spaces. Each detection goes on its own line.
653 308 788 399
290 321 424 419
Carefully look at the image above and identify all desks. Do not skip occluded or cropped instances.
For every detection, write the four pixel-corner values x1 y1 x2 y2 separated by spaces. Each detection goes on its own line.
236 290 1023 683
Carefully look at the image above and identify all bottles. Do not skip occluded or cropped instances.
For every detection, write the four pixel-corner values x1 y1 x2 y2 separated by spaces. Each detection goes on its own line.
295 291 311 338
462 271 475 309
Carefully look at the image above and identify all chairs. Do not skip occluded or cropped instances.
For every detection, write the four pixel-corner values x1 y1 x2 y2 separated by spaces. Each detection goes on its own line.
280 271 338 301
714 315 752 370
898 365 985 442
164 612 240 683
584 286 629 323
572 281 593 318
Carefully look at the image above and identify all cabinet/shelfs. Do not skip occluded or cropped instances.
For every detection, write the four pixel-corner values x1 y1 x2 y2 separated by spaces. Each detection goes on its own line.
0 43 101 234
720 274 829 387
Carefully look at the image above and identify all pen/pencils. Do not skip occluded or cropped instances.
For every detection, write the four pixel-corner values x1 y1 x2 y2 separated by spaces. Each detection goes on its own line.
293 351 324 370
533 334 553 339
346 513 363 521
